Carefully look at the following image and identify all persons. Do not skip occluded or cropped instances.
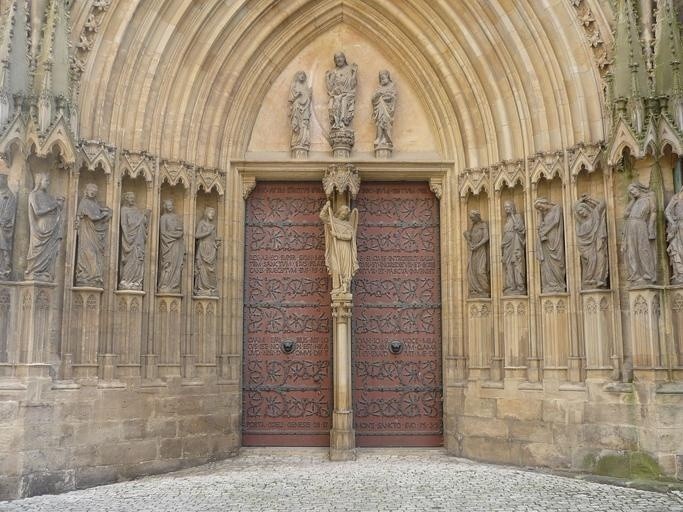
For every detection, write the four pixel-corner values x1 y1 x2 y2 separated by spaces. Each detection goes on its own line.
326 51 359 130
464 183 683 295
319 201 359 294
370 70 396 146
0 173 222 296
289 70 313 147
